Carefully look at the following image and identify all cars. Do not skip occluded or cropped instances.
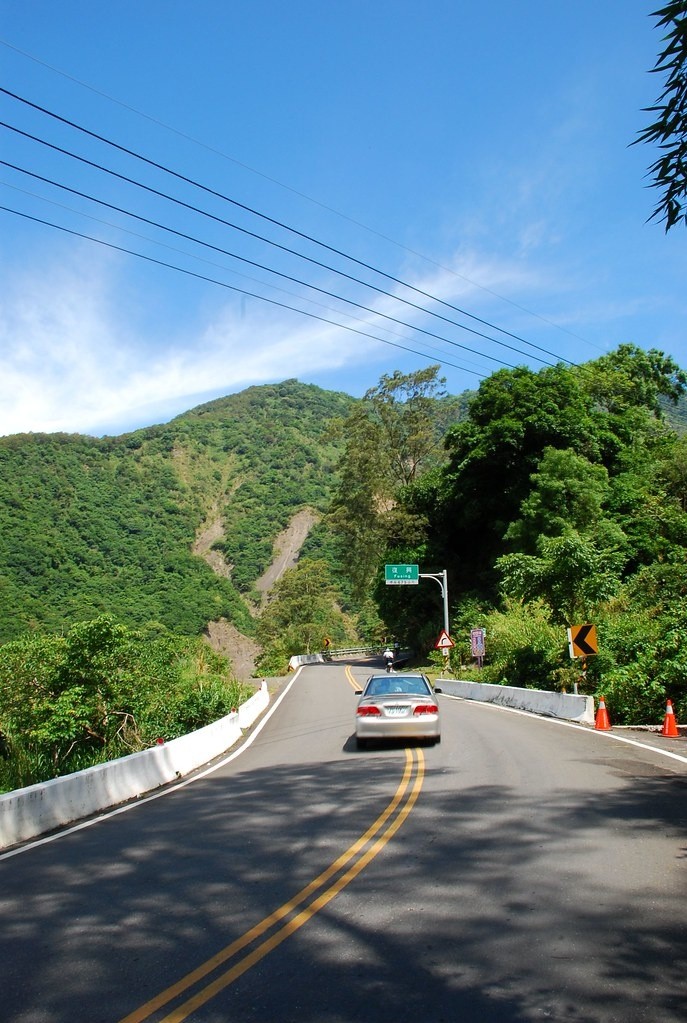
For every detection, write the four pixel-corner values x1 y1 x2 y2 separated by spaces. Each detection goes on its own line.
354 673 442 744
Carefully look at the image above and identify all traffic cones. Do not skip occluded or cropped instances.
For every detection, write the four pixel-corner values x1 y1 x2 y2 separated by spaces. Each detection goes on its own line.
657 699 682 738
592 697 614 731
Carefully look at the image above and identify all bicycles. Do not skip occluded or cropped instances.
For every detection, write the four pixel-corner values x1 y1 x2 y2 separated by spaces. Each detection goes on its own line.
385 658 394 673
395 648 399 657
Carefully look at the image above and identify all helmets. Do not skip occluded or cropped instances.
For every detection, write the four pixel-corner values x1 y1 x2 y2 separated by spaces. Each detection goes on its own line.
386 649 390 652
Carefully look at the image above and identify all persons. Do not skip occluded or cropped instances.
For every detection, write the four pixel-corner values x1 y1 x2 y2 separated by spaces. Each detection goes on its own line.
383 648 395 672
